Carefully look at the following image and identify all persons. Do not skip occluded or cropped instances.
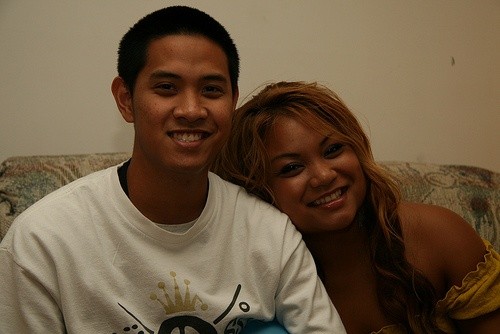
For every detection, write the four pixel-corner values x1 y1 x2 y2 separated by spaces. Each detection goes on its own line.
1 6 347 334
208 80 499 334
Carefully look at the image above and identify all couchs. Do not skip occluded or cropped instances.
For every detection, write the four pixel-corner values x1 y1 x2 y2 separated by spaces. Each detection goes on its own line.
0 149 499 264
